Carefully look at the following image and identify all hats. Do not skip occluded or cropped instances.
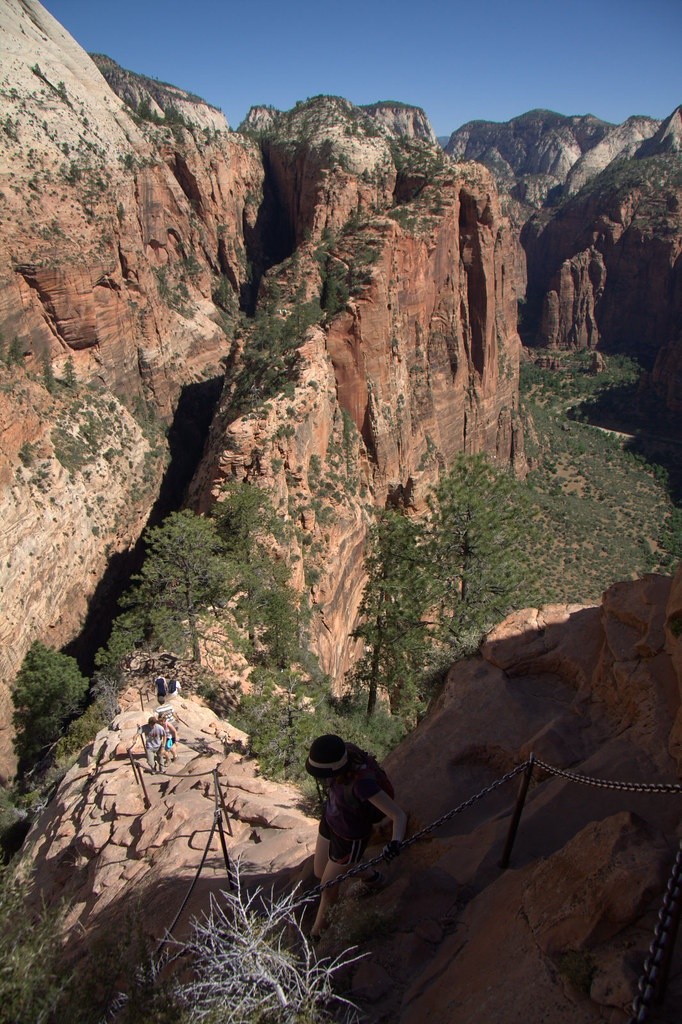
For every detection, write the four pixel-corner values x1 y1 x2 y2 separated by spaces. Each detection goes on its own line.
305 734 350 778
158 696 165 702
148 717 157 724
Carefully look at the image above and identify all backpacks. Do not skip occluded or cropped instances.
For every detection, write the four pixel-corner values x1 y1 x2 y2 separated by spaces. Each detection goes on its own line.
343 750 394 817
156 678 166 697
168 680 176 693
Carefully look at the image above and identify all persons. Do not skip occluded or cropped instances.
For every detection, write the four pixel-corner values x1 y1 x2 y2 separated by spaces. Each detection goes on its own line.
151 671 168 706
303 734 407 940
168 677 181 696
132 716 167 775
156 704 179 732
156 713 179 766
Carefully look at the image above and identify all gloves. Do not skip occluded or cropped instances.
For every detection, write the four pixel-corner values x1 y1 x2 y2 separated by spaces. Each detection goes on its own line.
382 840 402 863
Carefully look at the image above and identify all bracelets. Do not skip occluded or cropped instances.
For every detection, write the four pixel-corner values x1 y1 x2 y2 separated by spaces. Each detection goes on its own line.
161 745 165 747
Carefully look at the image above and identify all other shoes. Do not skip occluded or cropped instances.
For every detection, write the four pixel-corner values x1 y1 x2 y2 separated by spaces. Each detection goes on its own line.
164 759 171 767
173 755 179 761
151 769 155 775
160 766 163 773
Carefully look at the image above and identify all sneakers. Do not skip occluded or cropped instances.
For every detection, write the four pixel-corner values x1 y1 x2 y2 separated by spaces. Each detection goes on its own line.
299 933 320 958
